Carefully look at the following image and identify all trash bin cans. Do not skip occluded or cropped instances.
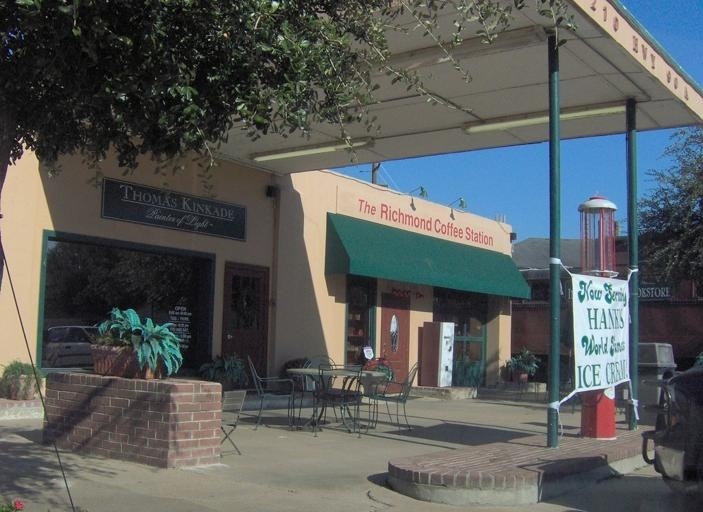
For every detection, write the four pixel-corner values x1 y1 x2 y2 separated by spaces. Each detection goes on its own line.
625 342 676 425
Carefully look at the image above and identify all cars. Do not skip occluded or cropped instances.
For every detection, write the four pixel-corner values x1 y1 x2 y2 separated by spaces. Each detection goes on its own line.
41 324 100 367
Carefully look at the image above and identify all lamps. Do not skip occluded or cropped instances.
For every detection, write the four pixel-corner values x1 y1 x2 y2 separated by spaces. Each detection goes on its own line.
447 196 467 210
408 184 428 200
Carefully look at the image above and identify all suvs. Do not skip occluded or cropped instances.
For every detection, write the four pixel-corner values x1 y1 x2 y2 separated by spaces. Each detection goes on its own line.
636 348 702 498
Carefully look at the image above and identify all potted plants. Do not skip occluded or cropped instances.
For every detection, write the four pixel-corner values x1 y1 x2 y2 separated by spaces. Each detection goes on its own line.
89 310 182 383
2 359 44 400
502 345 541 382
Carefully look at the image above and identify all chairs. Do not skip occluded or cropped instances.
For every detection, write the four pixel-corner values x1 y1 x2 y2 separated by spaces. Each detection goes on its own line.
215 385 246 457
241 347 423 440
